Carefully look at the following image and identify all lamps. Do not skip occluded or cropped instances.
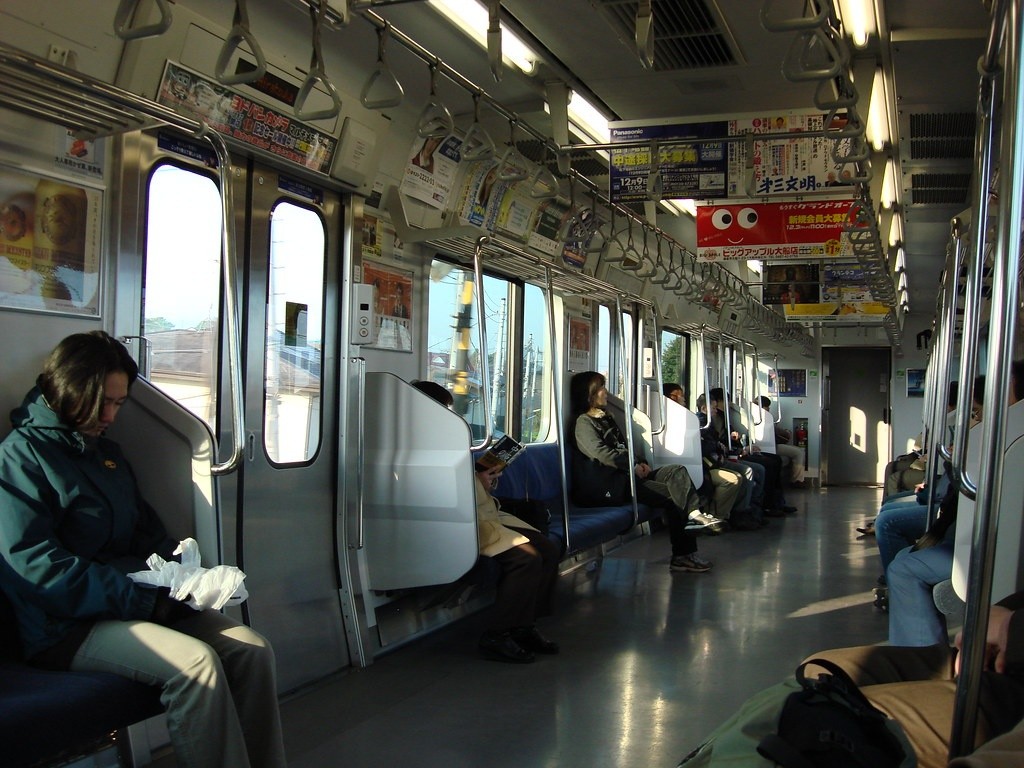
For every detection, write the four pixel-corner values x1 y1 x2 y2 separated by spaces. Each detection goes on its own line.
896 272 907 290
895 247 907 271
838 0 877 49
865 64 889 150
887 210 908 249
880 156 897 208
899 290 908 305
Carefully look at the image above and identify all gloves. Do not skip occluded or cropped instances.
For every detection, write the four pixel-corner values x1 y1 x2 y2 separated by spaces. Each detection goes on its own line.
149 586 202 630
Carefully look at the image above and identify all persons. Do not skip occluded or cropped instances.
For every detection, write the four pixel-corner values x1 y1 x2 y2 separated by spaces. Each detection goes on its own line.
781 285 800 304
406 380 560 662
412 126 447 174
825 171 855 187
478 163 504 209
0 331 287 768
662 361 1024 768
770 118 788 133
569 371 726 572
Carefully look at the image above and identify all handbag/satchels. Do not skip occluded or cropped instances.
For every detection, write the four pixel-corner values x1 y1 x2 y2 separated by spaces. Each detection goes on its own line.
582 485 624 508
493 496 552 537
757 656 918 768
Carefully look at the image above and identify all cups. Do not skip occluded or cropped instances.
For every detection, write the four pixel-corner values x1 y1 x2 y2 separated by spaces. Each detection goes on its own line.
728 456 737 462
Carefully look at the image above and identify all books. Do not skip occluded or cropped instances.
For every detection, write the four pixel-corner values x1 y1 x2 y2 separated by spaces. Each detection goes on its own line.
475 436 526 477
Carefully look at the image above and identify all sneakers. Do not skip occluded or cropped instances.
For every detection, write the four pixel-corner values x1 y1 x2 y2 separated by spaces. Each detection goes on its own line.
684 512 729 535
669 552 713 572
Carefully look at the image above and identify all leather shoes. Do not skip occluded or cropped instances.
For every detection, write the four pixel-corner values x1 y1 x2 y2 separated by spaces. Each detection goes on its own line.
477 631 536 663
511 624 560 655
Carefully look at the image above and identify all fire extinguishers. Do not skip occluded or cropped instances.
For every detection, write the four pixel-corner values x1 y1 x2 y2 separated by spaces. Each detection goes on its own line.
795 421 808 461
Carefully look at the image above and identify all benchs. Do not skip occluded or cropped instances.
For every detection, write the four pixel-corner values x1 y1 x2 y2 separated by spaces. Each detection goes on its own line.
475 444 659 565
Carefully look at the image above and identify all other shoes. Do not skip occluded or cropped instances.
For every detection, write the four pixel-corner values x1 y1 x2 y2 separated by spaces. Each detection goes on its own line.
856 528 876 535
775 504 798 513
740 510 770 525
762 507 783 517
729 510 759 530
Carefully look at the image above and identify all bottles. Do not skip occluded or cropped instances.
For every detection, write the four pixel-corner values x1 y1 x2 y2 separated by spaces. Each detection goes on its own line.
738 455 744 464
